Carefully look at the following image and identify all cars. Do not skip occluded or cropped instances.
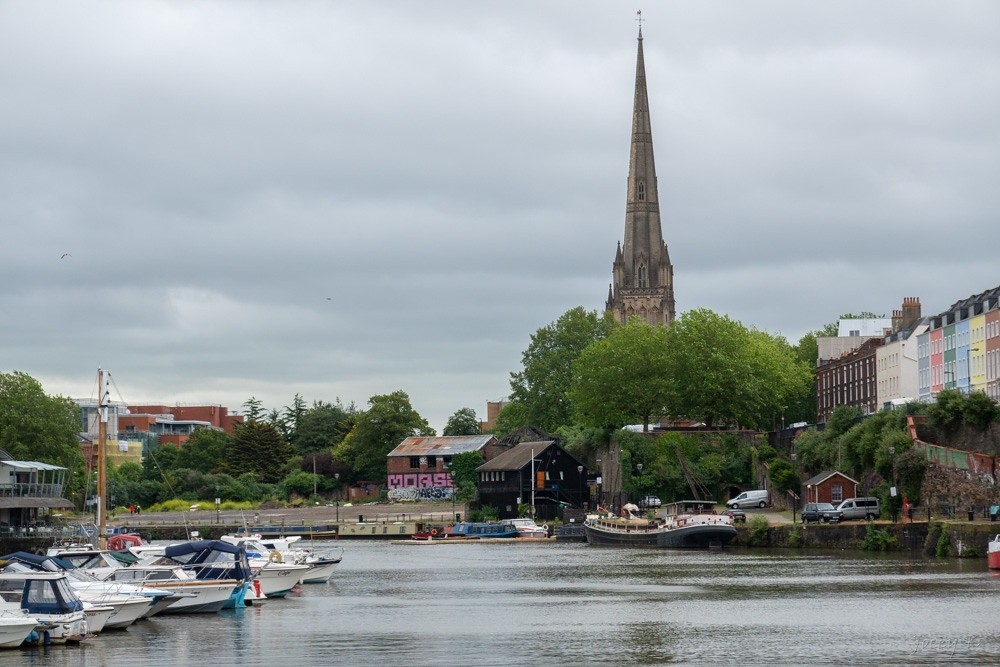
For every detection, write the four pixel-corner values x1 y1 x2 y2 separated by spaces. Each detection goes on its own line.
638 496 661 508
800 502 844 525
719 509 747 524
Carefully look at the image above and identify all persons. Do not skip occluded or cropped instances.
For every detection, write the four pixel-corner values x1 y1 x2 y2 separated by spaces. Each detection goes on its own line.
130 503 141 514
889 499 911 523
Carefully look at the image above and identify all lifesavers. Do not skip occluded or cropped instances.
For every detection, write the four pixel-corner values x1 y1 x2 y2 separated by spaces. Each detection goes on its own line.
542 524 548 530
269 552 282 562
431 529 437 535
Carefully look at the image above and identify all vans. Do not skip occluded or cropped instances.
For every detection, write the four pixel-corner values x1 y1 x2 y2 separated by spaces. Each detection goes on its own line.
725 489 770 510
836 496 881 523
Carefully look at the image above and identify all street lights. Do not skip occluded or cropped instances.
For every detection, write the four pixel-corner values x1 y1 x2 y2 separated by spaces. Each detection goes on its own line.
216 484 221 523
636 463 643 519
888 445 896 522
334 473 340 523
577 465 583 515
451 470 456 522
790 452 798 524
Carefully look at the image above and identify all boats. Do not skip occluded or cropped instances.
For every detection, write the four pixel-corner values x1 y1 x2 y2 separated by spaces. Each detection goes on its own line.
0 366 556 654
986 534 1000 571
580 499 738 550
